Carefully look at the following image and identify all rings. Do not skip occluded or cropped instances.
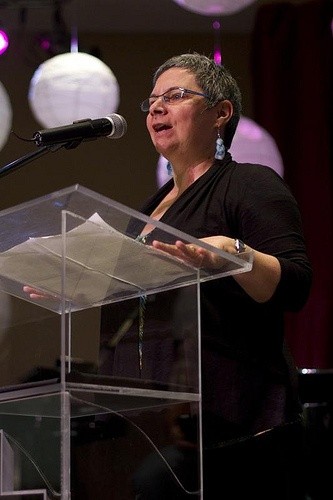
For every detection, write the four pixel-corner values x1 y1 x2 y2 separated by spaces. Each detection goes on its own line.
188 245 196 253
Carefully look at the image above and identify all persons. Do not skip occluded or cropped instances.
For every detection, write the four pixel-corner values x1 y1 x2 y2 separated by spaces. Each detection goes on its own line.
22 52 312 500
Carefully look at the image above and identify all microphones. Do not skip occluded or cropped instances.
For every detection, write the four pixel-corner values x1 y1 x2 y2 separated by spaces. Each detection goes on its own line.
33 113 127 146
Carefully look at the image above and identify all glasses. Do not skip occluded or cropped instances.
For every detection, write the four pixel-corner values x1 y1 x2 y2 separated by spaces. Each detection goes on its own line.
140 86 214 112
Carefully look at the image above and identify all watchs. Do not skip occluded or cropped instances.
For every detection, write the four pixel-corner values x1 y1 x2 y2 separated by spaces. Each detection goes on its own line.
235 239 245 258
